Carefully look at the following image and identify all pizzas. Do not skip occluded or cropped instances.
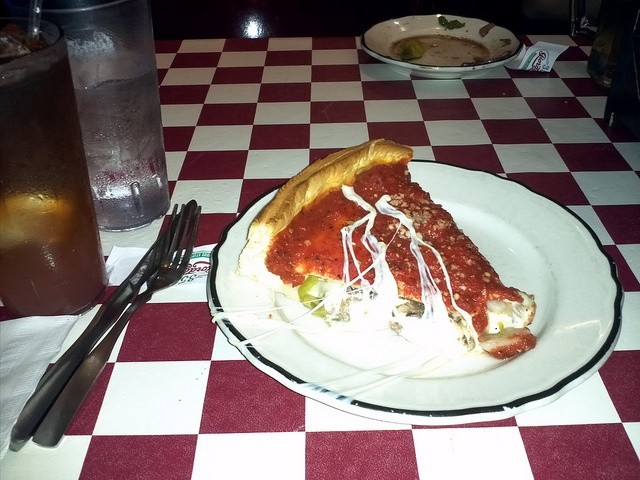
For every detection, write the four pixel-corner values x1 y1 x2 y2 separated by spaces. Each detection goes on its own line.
236 137 537 360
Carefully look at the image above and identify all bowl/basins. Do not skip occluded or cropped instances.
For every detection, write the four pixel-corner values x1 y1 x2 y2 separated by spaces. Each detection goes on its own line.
361 12 524 76
205 159 624 427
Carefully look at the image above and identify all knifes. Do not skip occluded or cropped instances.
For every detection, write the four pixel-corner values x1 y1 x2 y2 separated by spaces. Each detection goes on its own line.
11 201 197 444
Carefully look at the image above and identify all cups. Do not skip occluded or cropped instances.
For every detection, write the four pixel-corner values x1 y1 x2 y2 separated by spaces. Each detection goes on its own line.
1 14 108 316
8 1 170 230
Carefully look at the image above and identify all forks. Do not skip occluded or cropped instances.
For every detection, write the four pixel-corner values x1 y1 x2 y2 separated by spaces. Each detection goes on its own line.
34 203 203 448
